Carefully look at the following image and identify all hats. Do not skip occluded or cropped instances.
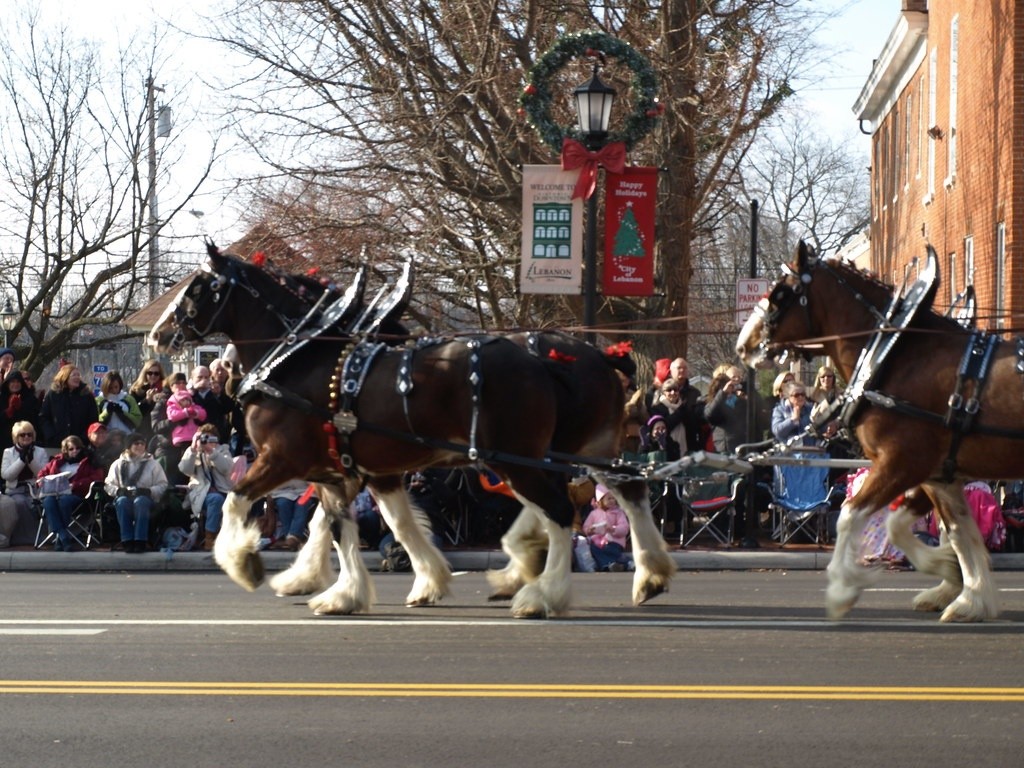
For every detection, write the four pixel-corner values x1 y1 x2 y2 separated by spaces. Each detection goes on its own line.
87 423 106 437
0 348 15 360
595 483 610 502
175 391 192 403
125 432 147 449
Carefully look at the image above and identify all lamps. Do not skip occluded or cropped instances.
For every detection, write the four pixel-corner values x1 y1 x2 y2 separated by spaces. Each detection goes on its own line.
927 125 942 139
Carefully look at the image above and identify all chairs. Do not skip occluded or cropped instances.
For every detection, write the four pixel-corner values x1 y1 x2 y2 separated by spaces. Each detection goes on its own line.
621 452 842 549
21 475 277 549
434 465 508 549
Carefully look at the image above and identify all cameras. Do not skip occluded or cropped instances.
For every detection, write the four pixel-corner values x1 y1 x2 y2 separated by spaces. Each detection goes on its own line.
200 434 218 444
127 486 138 495
207 382 213 387
733 384 744 390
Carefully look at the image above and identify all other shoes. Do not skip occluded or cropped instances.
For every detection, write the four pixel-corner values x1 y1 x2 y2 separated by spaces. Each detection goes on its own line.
54 532 372 554
608 560 635 572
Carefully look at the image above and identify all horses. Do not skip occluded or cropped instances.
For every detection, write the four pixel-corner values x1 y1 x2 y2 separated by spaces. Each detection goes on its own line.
150 238 680 620
734 239 1024 625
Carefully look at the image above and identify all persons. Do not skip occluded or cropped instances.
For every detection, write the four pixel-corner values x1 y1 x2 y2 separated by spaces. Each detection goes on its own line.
0 348 425 571
582 481 638 571
633 357 864 547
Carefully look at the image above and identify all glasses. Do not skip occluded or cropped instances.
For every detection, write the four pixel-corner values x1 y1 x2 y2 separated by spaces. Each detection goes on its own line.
147 371 160 376
791 392 805 397
20 433 33 438
664 388 678 393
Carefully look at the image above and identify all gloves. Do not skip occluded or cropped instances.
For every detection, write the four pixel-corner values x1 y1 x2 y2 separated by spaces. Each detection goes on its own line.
5 394 21 418
20 447 28 462
117 487 128 497
132 488 151 497
28 446 33 464
